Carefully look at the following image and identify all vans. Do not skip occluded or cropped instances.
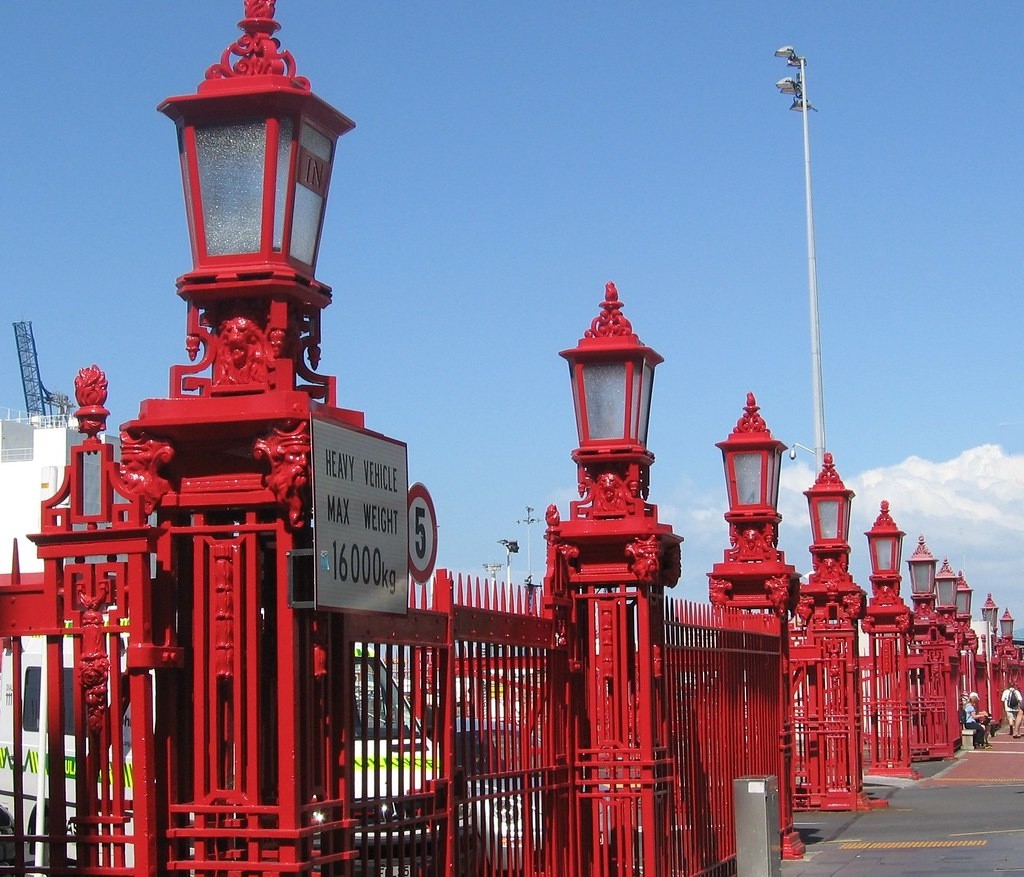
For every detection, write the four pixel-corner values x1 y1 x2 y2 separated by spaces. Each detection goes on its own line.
0 602 439 877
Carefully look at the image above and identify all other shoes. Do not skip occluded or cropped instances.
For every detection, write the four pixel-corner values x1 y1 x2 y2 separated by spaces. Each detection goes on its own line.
1013 733 1021 738
1008 730 1014 735
1019 732 1024 736
974 742 992 749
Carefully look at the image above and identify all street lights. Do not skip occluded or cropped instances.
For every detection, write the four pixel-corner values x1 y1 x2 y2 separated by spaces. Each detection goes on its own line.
773 43 823 478
497 539 520 612
483 563 502 610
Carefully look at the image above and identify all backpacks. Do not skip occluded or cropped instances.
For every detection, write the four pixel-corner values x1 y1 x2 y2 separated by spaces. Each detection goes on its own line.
959 705 973 724
1005 688 1020 708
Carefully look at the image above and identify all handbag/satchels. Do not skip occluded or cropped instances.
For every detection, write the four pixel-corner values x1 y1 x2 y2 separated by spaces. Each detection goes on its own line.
979 714 992 725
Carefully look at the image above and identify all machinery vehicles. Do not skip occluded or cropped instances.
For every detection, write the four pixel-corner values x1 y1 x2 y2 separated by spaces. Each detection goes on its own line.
11 320 77 428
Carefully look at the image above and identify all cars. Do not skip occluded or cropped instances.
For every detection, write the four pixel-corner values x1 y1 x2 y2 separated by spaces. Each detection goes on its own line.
454 716 613 872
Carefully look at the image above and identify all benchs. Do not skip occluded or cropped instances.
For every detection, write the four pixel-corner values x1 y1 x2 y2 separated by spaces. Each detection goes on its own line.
960 729 977 749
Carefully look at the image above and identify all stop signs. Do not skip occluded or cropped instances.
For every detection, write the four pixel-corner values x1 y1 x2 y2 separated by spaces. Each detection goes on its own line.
407 481 439 587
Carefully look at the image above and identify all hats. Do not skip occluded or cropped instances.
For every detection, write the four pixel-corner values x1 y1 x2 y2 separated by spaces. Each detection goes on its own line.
970 692 980 700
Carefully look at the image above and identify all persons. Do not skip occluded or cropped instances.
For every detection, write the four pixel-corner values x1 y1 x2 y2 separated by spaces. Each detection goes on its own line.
965 692 988 748
1001 682 1024 738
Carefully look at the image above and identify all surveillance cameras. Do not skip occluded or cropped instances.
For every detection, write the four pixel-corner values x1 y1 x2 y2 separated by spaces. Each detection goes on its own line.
789 449 796 460
